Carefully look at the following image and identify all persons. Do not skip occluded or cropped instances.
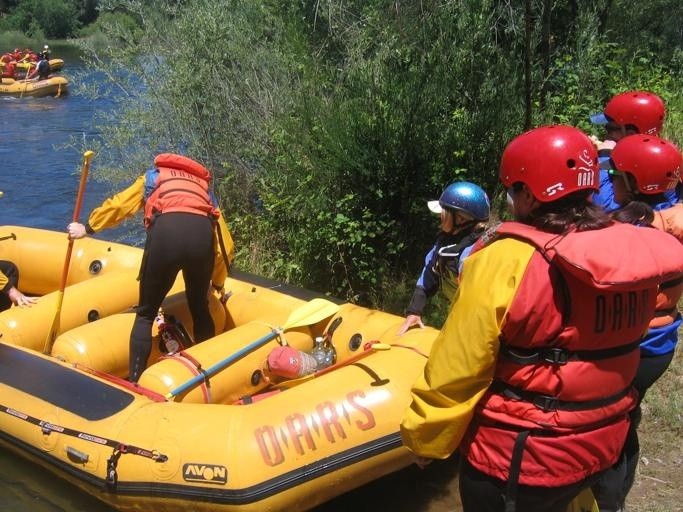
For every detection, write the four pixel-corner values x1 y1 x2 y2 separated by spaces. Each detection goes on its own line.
0 44 52 81
597 136 683 512
0 259 37 313
400 126 683 512
589 90 661 213
67 154 235 383
399 182 489 335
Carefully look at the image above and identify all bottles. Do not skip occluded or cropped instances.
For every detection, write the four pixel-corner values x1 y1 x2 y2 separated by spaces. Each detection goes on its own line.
169 315 196 349
155 317 184 355
323 334 338 369
310 335 326 372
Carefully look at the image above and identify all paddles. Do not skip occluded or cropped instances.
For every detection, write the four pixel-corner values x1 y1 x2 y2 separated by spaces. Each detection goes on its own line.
165 298 340 399
45 151 96 355
20 64 30 98
269 343 392 390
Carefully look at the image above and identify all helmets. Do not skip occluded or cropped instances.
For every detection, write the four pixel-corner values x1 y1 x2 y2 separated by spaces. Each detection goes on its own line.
501 123 600 200
609 133 682 196
605 89 663 133
428 180 489 223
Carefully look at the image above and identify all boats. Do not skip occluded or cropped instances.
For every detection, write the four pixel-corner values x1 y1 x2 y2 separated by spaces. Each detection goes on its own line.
0 58 63 75
0 74 68 99
0 224 441 512
